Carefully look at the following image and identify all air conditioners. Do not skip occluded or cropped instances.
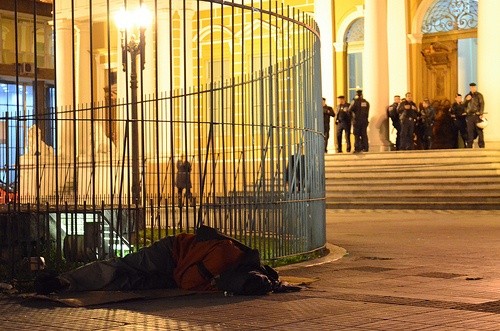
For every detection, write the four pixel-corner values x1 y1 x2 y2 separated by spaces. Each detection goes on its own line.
20 62 35 76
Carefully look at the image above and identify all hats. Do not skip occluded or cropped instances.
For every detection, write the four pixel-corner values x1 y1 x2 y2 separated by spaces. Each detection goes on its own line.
469 83 476 86
338 96 344 98
356 90 362 93
455 93 461 97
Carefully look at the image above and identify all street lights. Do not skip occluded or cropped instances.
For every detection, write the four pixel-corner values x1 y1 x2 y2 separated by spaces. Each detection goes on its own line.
111 3 154 230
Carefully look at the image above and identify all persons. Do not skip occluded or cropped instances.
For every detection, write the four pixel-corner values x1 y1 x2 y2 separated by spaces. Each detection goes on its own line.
335 90 370 153
387 82 485 151
35 233 273 295
176 152 193 208
322 97 336 153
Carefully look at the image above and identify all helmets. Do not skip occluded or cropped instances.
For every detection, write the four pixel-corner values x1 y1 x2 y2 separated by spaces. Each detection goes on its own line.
476 118 489 129
